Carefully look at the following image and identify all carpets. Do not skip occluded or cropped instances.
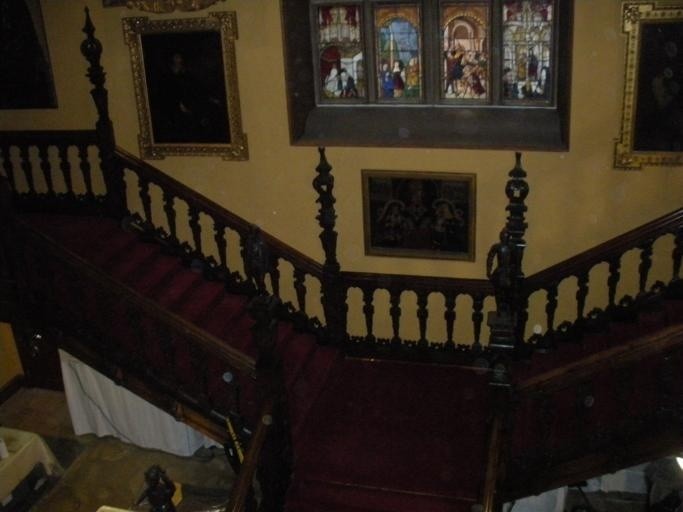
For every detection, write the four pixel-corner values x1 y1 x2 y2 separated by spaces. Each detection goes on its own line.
0 430 83 510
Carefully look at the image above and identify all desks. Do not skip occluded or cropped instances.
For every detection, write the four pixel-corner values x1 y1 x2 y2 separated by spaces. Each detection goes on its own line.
0 425 59 500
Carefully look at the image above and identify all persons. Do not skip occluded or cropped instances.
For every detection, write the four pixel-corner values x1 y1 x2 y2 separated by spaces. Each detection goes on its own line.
382 59 408 101
375 197 413 251
444 38 488 99
429 200 468 254
337 67 359 99
524 48 540 84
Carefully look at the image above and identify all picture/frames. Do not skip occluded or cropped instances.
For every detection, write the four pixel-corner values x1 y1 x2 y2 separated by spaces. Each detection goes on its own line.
122 11 248 163
277 0 574 154
613 0 682 172
358 166 477 263
